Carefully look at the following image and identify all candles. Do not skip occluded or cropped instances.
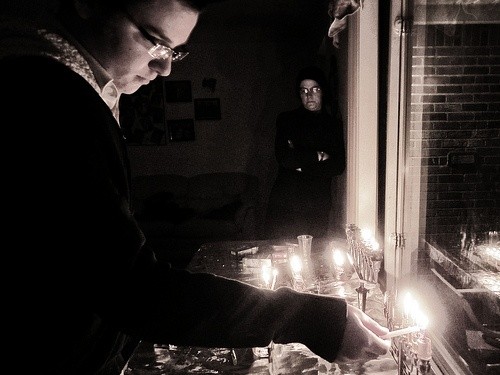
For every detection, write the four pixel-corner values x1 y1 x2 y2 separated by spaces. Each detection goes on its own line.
379 315 428 340
271 268 279 290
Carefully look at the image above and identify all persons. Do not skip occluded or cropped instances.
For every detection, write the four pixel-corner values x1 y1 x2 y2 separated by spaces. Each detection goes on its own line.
275 74 347 240
0 0 392 375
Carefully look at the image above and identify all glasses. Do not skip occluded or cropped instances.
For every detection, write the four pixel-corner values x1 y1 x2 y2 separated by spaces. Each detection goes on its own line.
114 0 190 63
300 87 321 94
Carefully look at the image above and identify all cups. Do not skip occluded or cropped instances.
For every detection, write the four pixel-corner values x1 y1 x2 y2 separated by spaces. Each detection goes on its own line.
297 235 313 258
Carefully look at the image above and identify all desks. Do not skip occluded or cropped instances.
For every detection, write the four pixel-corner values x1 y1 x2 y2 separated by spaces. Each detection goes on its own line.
117 241 443 375
